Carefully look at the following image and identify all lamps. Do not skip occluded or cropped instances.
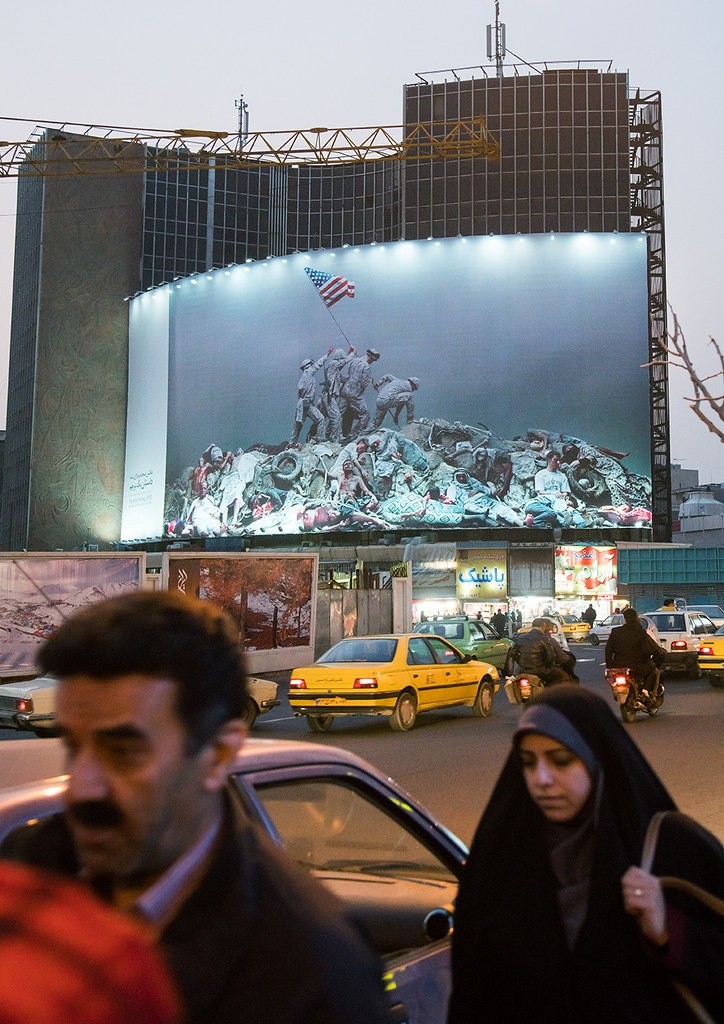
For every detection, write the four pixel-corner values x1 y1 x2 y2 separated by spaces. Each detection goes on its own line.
489 229 647 237
401 523 406 527
299 526 306 533
201 532 207 537
316 525 321 530
278 526 284 533
244 529 249 534
545 523 551 529
214 532 220 536
260 528 266 533
424 523 429 527
245 232 463 263
337 527 344 533
109 536 163 545
642 520 648 526
227 530 235 536
123 262 239 302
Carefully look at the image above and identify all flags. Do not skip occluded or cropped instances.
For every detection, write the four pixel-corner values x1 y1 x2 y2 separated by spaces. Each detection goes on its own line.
305 266 356 309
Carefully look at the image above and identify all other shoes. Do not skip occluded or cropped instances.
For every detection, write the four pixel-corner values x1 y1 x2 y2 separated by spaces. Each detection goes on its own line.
641 689 650 699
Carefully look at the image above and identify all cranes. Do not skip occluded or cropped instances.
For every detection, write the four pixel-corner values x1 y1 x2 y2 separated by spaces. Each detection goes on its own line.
0 111 501 178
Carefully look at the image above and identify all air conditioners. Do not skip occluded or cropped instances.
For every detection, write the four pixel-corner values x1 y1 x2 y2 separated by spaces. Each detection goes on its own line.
320 541 333 547
89 545 99 551
301 540 315 547
174 542 192 548
168 545 180 549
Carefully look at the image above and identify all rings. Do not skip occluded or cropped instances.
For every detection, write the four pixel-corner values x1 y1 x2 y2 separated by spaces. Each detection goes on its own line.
635 889 641 896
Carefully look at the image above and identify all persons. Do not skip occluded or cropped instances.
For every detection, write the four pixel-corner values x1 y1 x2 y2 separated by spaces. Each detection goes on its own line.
420 598 678 680
0 592 390 1024
448 681 724 1024
605 609 657 697
511 619 561 683
164 345 652 537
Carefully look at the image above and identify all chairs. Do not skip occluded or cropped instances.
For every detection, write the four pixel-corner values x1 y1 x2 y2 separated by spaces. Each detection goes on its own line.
457 625 463 636
435 627 445 638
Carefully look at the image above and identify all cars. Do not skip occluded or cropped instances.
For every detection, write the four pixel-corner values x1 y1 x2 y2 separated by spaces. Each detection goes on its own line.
638 604 724 689
0 733 470 1024
514 609 592 643
588 613 624 646
0 671 282 737
288 631 502 733
406 614 516 678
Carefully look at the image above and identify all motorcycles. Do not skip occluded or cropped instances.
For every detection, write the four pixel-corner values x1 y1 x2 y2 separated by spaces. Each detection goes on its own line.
511 654 576 716
603 651 671 724
